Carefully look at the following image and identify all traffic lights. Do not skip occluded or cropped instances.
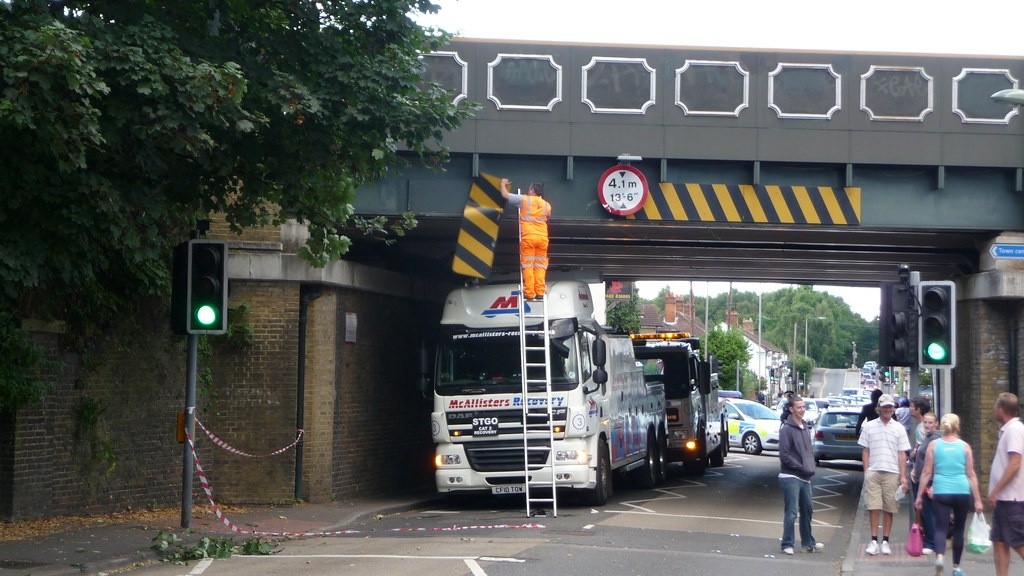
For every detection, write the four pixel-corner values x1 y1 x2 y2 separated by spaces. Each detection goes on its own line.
186 238 229 335
917 280 958 369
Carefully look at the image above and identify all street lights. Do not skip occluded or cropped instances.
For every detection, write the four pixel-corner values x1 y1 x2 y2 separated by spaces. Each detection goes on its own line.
803 316 828 398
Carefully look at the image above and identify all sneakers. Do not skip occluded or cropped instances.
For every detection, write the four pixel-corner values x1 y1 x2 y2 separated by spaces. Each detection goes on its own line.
783 548 794 555
800 543 824 553
880 541 891 554
865 540 879 555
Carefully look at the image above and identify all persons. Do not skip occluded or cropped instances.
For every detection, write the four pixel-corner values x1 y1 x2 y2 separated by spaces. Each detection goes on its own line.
916 413 983 576
856 389 942 554
988 393 1024 576
779 397 825 553
501 179 552 299
759 387 789 411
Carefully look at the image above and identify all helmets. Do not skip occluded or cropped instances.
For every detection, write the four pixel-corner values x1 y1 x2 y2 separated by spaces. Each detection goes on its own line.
897 397 908 407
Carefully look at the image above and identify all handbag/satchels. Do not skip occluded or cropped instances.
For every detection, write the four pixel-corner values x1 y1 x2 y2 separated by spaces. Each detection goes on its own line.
964 511 992 554
905 507 923 557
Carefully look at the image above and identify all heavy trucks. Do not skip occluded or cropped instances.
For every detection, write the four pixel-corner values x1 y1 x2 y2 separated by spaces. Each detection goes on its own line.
629 331 730 480
419 276 671 509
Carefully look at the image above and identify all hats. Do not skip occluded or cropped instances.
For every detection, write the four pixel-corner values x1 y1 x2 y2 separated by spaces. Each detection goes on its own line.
878 394 894 407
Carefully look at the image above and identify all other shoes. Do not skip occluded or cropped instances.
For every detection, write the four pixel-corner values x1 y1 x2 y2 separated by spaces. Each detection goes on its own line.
953 568 966 576
921 546 937 555
934 560 943 576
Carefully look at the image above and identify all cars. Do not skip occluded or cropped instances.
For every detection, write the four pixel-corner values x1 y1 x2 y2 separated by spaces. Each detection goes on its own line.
716 390 783 456
773 360 901 467
